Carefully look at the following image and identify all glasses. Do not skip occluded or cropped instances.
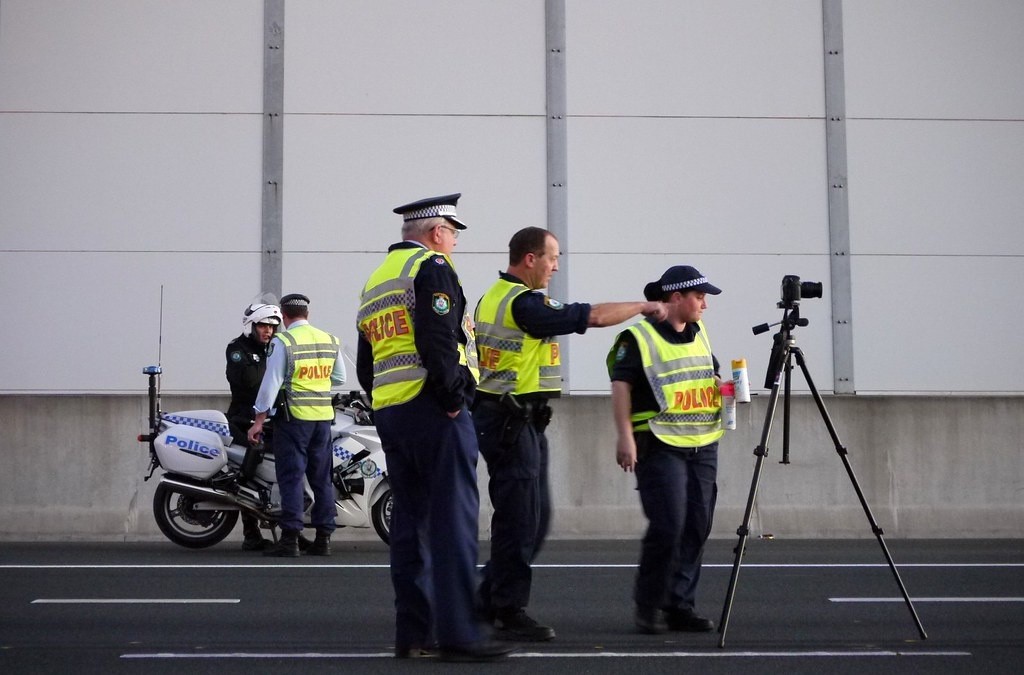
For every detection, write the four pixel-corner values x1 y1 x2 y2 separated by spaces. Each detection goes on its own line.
429 225 460 238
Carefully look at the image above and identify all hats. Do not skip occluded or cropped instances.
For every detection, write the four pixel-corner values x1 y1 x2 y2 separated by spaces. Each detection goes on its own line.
280 294 310 307
660 265 722 295
392 192 467 231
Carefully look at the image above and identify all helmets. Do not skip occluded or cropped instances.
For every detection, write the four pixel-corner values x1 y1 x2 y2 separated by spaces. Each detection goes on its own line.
241 302 283 338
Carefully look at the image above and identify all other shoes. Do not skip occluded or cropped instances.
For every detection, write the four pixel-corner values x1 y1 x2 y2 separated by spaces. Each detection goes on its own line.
667 609 713 634
394 639 445 658
441 635 520 661
634 604 668 632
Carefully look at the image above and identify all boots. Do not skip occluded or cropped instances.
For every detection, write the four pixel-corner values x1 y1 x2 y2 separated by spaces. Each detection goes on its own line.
236 440 265 492
240 508 274 550
307 529 332 556
263 530 300 558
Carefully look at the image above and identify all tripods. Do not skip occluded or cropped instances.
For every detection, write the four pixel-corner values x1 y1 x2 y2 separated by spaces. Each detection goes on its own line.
716 300 929 649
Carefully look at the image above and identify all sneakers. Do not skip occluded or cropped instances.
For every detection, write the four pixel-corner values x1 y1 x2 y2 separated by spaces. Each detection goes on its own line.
482 604 556 643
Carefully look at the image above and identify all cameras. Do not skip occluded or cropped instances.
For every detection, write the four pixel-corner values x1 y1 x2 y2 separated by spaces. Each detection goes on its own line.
780 275 822 301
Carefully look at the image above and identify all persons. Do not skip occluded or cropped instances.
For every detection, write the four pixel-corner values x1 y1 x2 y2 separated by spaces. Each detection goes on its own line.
355 194 520 659
470 227 678 642
249 291 345 556
605 266 752 635
226 301 310 552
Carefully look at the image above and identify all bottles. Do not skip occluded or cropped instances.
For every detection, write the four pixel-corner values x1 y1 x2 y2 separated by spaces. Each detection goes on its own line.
731 359 752 403
720 385 736 430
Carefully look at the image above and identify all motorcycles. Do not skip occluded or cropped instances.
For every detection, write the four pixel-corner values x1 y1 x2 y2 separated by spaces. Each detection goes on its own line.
138 284 393 547
